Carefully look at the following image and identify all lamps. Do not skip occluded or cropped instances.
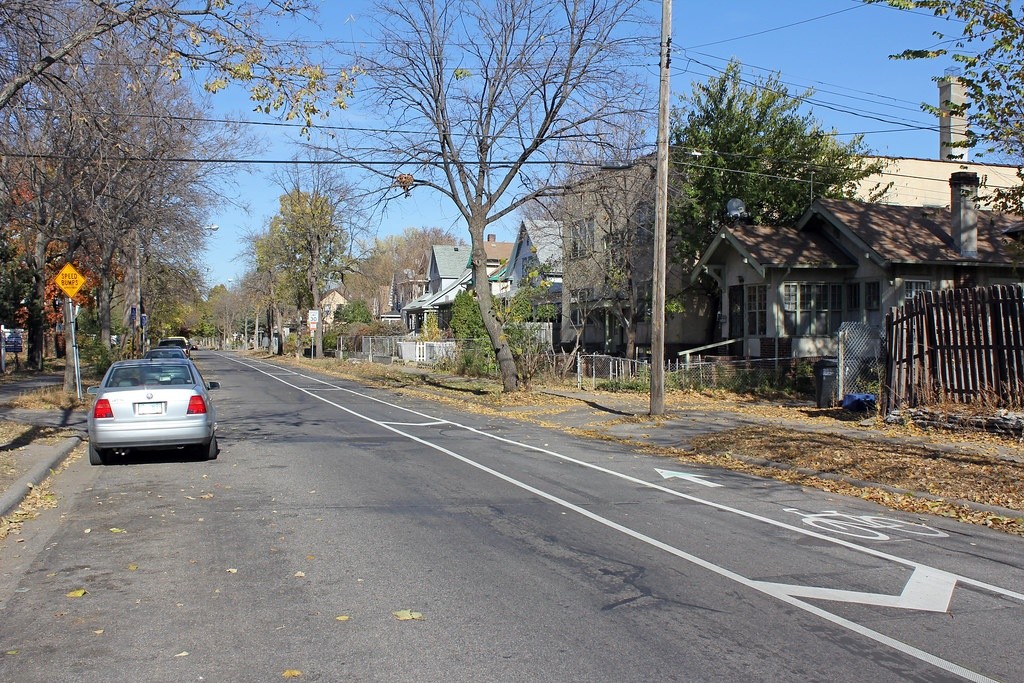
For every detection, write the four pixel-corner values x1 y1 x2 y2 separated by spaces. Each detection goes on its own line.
717 311 727 323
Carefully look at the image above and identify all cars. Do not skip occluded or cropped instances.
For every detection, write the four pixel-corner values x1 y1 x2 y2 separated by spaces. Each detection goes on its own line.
191 345 198 351
157 336 192 350
87 358 221 464
141 348 190 383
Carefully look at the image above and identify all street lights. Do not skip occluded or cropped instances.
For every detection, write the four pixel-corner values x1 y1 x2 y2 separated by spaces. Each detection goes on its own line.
133 225 220 358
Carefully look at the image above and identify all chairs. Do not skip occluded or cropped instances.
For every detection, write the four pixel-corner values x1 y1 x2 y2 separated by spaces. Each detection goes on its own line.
171 377 186 385
145 379 160 385
118 378 139 387
172 354 181 357
156 354 162 357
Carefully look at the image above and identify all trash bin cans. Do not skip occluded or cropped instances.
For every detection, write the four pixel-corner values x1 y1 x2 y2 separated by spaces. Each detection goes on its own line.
813 359 861 409
